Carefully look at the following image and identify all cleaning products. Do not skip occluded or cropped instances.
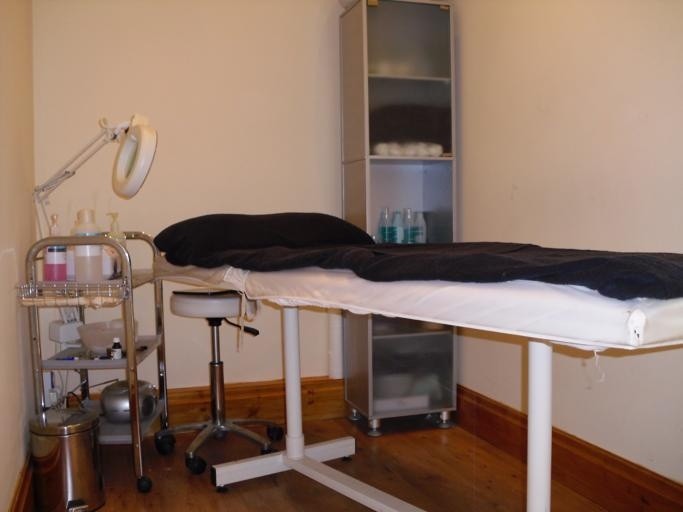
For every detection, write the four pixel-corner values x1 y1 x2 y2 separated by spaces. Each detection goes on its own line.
379 206 426 245
71 210 102 281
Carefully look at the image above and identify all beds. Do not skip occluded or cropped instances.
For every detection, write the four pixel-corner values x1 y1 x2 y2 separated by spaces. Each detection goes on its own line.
156 243 683 512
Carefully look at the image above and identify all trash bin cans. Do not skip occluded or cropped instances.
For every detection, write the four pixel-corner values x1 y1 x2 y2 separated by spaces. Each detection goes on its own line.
29 407 105 512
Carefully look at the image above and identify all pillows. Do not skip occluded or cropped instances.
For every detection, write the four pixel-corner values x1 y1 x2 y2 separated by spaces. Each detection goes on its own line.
155 213 374 266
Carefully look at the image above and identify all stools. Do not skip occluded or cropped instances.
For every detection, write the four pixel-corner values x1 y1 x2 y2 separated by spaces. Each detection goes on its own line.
159 288 285 475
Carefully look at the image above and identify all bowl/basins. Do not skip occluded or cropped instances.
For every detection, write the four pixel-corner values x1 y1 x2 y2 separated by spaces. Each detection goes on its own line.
76 319 137 354
375 372 413 397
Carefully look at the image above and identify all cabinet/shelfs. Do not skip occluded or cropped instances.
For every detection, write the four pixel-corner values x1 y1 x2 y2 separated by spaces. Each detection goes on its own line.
18 232 171 492
340 0 459 434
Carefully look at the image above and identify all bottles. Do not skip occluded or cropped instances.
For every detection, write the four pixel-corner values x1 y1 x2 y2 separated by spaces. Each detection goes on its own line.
379 208 427 246
110 337 123 361
73 208 104 286
